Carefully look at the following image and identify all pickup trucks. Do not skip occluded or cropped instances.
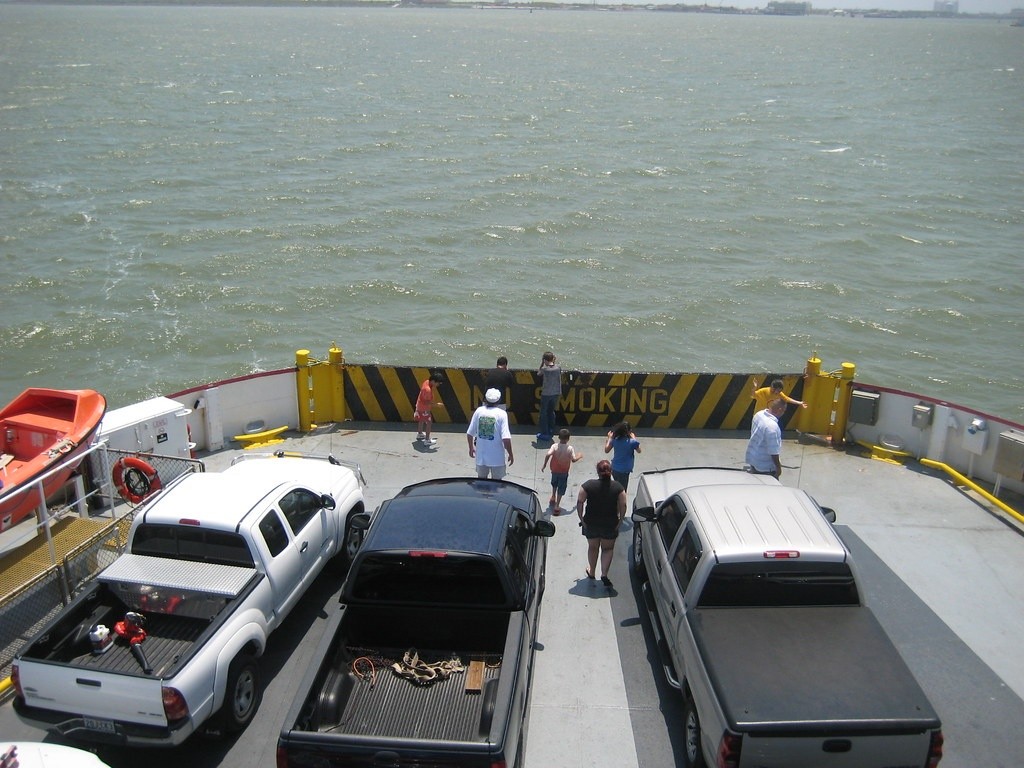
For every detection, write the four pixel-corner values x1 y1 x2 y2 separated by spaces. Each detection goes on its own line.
11 451 368 747
274 476 557 768
629 465 945 768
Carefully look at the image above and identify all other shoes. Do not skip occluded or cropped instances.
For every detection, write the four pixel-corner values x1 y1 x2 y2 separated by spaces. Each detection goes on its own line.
550 497 555 502
537 433 551 441
553 507 561 515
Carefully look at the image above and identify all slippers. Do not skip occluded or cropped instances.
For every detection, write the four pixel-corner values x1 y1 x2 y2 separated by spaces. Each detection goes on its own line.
601 576 612 586
586 568 595 579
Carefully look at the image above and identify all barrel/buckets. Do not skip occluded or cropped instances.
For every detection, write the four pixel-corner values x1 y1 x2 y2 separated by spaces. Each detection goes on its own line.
89 625 113 653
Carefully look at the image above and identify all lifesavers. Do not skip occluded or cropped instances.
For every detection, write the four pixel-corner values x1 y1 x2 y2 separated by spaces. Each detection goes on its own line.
113 457 162 504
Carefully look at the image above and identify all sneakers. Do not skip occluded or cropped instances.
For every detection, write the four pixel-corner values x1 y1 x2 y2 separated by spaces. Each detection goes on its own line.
416 433 426 439
424 439 437 446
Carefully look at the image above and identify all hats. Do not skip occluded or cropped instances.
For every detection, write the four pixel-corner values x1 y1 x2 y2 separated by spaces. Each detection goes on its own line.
485 388 501 403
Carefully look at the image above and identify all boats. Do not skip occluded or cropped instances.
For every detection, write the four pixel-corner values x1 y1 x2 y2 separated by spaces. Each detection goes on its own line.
0 387 107 533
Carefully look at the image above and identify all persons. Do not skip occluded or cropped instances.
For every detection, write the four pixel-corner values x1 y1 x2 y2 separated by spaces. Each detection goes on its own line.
745 398 788 481
541 428 583 513
604 421 642 516
749 377 807 419
537 351 562 440
576 460 627 586
482 357 517 411
467 388 514 480
413 372 445 447
